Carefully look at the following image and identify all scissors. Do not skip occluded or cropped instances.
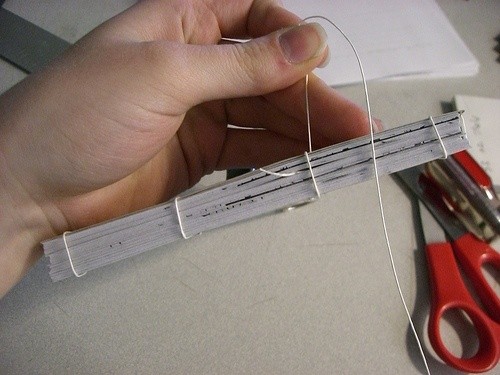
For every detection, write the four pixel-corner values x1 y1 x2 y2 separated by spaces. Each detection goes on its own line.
395 164 500 372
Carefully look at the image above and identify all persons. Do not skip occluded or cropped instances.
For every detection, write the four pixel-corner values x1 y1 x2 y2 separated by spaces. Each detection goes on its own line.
0 1 383 302
